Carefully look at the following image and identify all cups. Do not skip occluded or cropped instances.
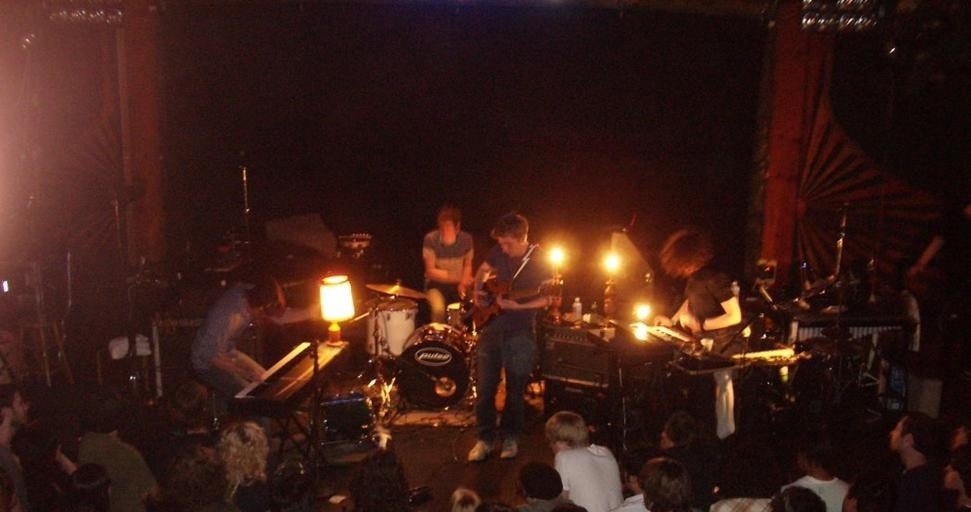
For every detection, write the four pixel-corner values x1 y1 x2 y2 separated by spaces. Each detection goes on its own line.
701 338 713 351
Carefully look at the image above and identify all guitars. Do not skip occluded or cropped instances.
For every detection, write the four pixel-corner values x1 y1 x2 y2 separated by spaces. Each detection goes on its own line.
462 278 567 327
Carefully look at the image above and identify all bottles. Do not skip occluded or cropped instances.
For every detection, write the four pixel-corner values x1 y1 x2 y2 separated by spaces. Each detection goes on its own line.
572 297 582 320
590 301 598 324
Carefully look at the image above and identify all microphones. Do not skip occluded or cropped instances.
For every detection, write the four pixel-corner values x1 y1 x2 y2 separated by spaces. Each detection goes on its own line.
762 286 802 304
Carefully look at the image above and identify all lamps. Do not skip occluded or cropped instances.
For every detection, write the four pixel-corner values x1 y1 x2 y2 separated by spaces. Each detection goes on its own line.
541 238 573 325
598 246 629 319
318 273 357 347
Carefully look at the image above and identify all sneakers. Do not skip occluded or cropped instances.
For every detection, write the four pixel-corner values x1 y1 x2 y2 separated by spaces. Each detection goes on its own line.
500 438 518 458
467 440 496 461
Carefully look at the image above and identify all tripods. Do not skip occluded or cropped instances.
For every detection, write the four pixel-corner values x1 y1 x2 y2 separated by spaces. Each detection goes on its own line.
301 337 351 505
793 359 868 423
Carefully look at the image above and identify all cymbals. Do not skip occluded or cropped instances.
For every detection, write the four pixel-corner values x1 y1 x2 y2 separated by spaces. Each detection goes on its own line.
366 280 425 300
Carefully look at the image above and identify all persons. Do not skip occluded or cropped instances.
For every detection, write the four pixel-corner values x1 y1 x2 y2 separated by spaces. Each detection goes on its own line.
422 205 971 512
1 279 411 512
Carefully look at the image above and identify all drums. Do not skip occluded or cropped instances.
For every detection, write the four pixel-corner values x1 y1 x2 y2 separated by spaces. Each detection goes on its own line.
396 322 471 410
366 299 417 358
447 302 464 326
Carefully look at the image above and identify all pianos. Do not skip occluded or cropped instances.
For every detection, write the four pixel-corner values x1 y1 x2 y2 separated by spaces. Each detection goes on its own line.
646 324 696 349
233 338 350 411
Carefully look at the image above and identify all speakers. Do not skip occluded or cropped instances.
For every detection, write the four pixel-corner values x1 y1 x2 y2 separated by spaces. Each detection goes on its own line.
543 374 616 425
